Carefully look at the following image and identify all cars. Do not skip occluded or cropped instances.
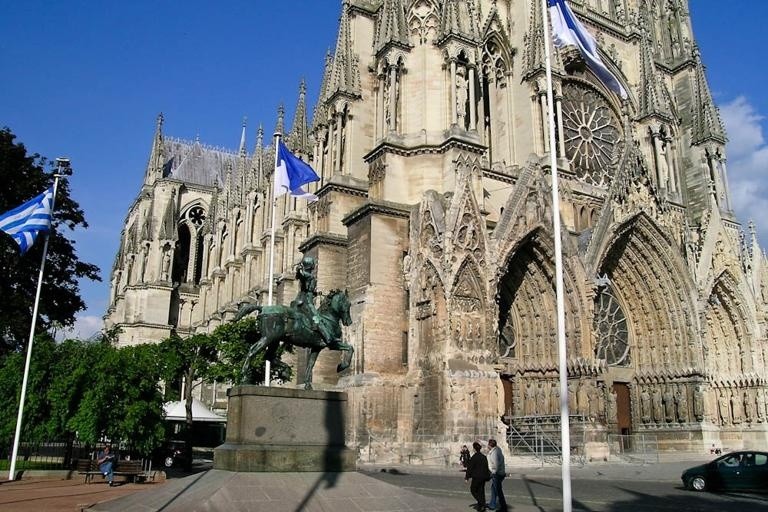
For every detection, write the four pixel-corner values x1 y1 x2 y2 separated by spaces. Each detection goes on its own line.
157 440 186 468
681 450 767 492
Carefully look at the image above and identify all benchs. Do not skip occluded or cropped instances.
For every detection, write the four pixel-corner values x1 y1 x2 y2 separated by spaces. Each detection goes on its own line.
76 460 141 485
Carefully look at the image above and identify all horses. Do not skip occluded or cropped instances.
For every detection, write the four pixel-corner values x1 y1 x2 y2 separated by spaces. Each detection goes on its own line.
230 287 355 390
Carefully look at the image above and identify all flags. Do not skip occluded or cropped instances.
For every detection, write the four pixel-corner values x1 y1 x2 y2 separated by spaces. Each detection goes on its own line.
0 184 56 256
547 0 628 100
274 139 320 202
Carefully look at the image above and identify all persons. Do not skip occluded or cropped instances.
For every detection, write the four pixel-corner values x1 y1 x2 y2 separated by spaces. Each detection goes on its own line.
97 444 114 487
487 439 509 510
459 445 470 467
710 444 716 454
292 257 341 344
465 442 492 512
